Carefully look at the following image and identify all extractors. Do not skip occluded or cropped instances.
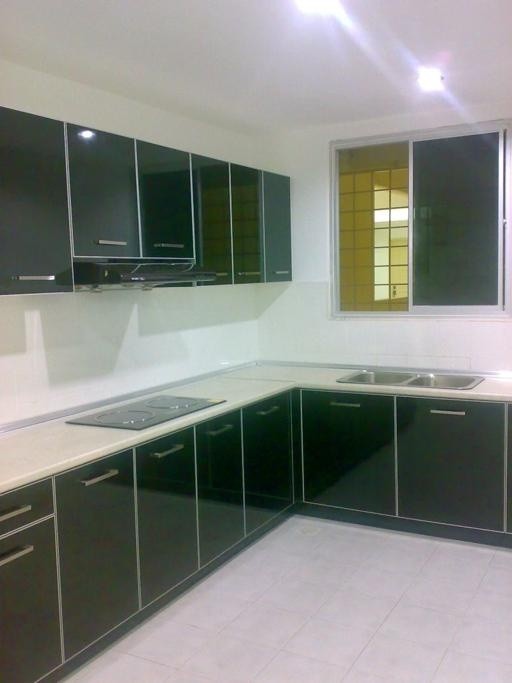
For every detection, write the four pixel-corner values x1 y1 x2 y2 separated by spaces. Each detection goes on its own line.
71 258 196 294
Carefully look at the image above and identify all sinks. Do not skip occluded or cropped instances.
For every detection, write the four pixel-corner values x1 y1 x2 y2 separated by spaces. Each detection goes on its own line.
404 373 485 390
336 369 412 386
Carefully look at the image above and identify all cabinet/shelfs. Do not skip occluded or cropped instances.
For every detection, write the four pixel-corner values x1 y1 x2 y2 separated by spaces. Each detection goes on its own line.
241 388 296 538
0 107 74 294
64 121 141 261
190 153 234 285
229 163 266 285
299 384 397 523
52 448 139 663
193 409 246 570
398 392 508 541
262 171 294 283
135 139 197 263
133 426 198 609
0 477 63 683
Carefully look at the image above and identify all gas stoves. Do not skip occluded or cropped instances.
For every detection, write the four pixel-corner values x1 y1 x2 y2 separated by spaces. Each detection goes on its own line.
68 394 226 432
